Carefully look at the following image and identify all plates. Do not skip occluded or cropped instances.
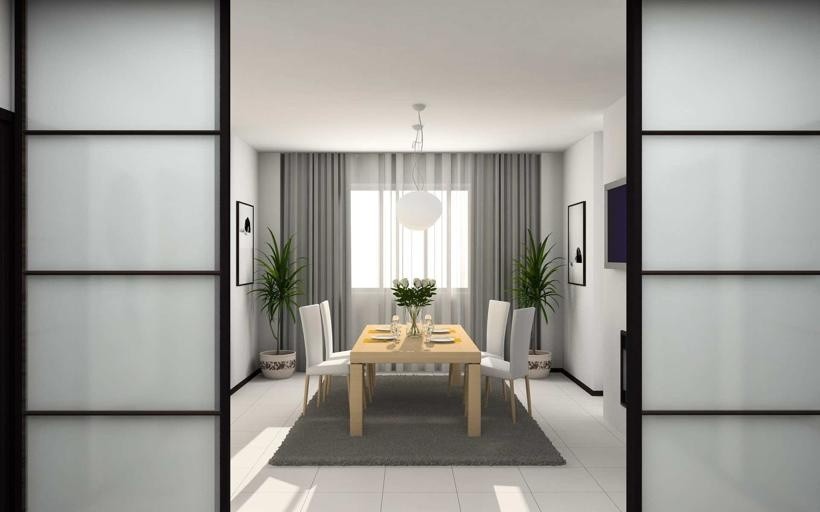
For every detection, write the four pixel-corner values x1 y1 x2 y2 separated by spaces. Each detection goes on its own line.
431 339 454 342
376 327 391 330
432 330 448 333
370 334 396 340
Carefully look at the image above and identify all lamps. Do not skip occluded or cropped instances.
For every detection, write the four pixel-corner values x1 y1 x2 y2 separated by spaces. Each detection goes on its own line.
397 103 443 231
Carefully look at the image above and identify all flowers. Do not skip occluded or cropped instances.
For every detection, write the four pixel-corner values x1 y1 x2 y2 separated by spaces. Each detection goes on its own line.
390 277 437 334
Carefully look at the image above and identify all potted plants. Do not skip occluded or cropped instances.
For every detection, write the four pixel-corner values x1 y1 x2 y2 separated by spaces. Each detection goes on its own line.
247 226 310 379
502 229 566 379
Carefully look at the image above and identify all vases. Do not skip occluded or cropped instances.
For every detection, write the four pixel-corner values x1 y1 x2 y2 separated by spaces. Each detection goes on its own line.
406 307 422 336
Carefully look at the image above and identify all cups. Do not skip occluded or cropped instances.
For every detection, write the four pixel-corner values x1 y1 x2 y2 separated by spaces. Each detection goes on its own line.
423 314 432 342
391 315 401 343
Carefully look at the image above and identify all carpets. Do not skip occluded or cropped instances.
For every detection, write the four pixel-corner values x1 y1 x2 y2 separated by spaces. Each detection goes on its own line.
268 374 567 466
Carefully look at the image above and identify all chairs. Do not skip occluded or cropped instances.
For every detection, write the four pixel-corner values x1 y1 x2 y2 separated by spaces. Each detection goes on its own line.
320 300 375 401
481 299 510 393
298 304 367 416
464 307 536 423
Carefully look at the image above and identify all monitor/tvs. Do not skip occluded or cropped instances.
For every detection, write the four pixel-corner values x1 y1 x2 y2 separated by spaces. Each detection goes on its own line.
604 176 627 269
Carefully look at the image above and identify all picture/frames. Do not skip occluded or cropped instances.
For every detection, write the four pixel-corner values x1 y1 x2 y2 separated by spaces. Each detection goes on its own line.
567 200 586 287
236 201 254 286
